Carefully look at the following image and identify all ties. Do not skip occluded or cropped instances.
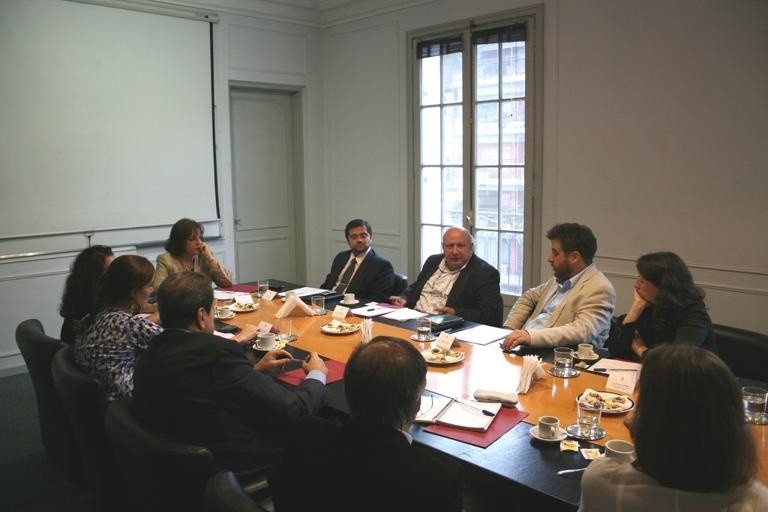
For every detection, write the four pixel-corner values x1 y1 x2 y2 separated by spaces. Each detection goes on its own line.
336 258 357 293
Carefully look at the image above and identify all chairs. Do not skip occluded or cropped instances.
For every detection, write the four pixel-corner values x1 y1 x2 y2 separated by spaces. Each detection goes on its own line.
595 316 627 359
102 395 215 512
200 468 274 510
708 322 767 385
15 317 78 444
390 271 410 300
50 344 109 443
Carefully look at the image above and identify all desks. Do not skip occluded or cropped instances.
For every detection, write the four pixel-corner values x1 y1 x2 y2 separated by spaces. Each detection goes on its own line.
132 275 768 509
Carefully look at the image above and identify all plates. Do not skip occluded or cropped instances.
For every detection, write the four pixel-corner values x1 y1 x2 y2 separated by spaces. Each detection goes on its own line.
319 322 359 334
214 310 235 320
530 425 568 441
577 390 635 414
566 423 606 441
420 348 464 365
252 341 287 352
570 351 600 360
410 333 436 342
549 367 580 380
227 303 259 312
339 299 360 305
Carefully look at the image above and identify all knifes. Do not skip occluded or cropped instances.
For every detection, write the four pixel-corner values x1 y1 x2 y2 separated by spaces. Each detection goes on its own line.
454 399 495 418
593 366 638 372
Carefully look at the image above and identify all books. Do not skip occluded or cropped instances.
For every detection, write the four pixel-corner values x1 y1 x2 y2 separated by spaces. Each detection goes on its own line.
420 313 462 331
407 390 505 434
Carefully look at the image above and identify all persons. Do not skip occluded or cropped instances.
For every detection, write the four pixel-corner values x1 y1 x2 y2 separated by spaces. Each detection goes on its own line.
59 243 163 343
68 254 260 403
384 226 504 327
150 218 235 303
268 335 471 512
573 339 768 512
498 221 618 352
605 250 720 369
318 217 395 302
129 270 326 485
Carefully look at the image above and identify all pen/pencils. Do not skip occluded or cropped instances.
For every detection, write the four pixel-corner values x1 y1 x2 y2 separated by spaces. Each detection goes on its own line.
367 307 382 311
594 368 637 371
454 400 495 416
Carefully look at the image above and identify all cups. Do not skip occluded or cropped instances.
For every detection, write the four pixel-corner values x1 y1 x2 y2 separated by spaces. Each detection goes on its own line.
343 293 355 303
577 401 602 434
578 342 596 357
257 280 269 296
741 386 767 423
553 347 574 374
538 415 560 438
604 440 635 462
311 295 325 314
416 317 432 339
255 333 276 349
277 317 292 340
